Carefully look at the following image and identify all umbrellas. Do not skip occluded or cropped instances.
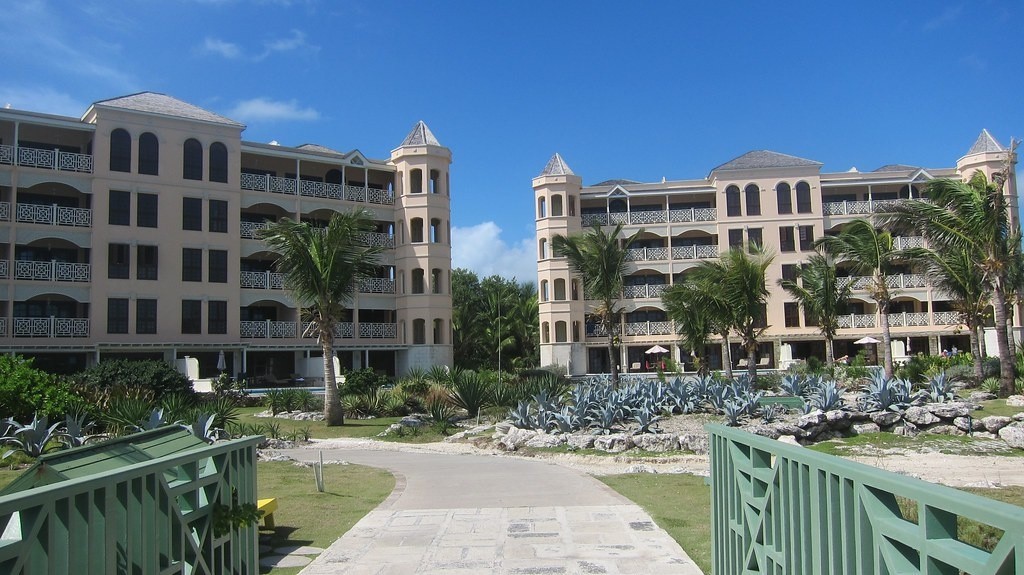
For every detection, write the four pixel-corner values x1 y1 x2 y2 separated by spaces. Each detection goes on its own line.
854 336 881 351
217 350 226 374
645 345 669 362
907 336 911 356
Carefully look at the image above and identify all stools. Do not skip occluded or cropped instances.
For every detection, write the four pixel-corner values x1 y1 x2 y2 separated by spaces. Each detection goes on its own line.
645 361 652 371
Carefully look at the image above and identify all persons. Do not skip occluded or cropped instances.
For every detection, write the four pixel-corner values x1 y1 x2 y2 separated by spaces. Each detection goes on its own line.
941 350 948 357
952 345 957 355
843 355 851 366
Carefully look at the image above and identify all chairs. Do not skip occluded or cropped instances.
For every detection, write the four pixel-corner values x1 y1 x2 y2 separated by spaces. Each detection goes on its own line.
661 362 666 372
291 373 316 387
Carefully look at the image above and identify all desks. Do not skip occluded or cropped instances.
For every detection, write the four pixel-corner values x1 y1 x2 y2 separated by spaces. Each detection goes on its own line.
651 362 659 371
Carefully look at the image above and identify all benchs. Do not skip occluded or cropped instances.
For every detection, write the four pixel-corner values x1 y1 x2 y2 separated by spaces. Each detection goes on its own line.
755 358 770 369
736 359 749 369
630 363 641 372
